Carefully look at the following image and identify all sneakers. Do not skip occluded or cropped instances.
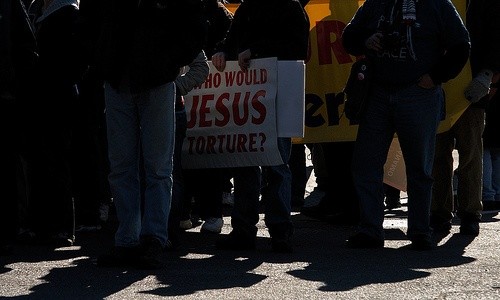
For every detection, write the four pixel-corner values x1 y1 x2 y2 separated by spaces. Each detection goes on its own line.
179 219 193 229
202 217 222 234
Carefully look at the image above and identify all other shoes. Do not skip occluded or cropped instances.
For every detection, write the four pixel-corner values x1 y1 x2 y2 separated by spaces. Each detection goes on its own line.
483 201 500 211
366 237 384 249
412 237 435 251
141 238 161 260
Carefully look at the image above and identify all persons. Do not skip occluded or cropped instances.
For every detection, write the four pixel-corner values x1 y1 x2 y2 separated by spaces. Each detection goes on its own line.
0 0 500 269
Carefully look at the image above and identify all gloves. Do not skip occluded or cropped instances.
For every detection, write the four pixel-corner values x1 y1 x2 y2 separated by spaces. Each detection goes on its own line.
463 70 494 103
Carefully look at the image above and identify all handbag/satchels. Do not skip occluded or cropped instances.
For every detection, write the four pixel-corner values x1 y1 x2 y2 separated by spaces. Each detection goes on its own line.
343 57 368 125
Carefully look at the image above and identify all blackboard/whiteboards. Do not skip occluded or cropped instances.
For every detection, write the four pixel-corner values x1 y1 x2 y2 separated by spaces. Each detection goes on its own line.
276 60 305 138
